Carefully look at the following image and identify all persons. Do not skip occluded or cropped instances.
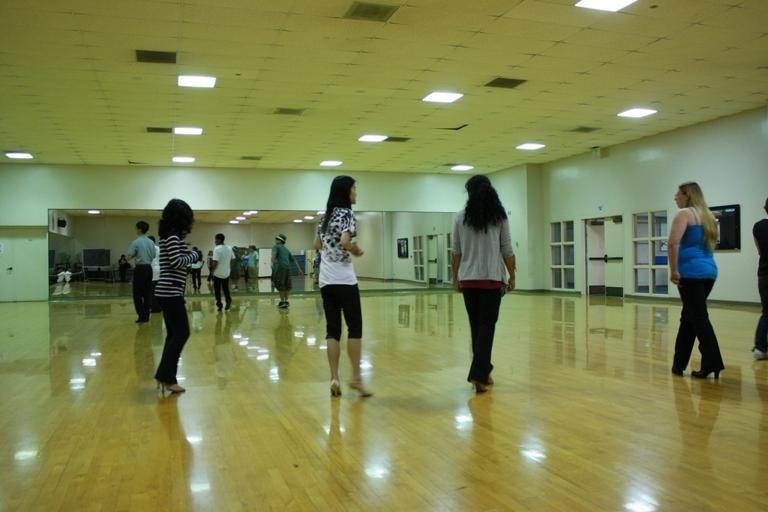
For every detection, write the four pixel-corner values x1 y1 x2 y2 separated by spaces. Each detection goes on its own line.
210 307 249 395
188 298 206 333
312 248 319 285
210 232 236 312
187 247 214 290
450 174 517 394
270 235 304 309
752 198 767 361
273 307 307 381
315 176 373 397
126 219 157 323
119 254 131 282
667 182 725 379
231 245 258 290
153 198 202 396
148 236 163 312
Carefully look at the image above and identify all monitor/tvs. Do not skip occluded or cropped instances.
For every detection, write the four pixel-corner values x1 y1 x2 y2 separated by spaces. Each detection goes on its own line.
709 205 740 250
397 238 408 257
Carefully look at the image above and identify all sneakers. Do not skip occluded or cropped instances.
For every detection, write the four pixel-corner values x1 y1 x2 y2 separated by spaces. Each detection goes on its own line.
225 303 230 310
218 307 222 311
753 349 768 359
285 301 289 308
278 302 284 307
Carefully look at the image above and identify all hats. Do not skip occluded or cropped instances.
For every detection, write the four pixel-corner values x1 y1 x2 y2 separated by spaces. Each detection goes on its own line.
275 234 287 243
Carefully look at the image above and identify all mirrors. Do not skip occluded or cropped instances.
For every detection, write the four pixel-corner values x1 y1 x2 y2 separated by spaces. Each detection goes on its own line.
47 208 458 300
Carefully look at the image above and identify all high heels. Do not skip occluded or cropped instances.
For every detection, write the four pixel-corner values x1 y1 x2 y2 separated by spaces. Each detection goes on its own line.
348 382 375 396
470 380 488 392
162 383 185 395
330 380 341 397
692 370 720 379
